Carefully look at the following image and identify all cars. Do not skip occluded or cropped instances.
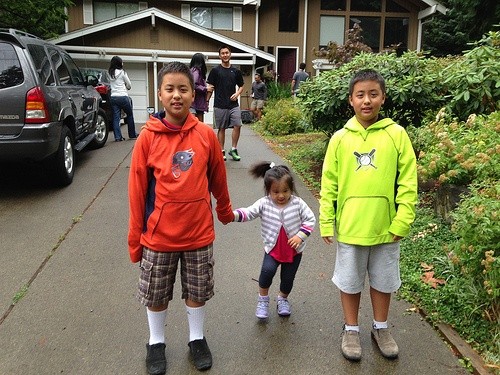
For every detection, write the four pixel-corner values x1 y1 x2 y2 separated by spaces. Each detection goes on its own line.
79 67 133 132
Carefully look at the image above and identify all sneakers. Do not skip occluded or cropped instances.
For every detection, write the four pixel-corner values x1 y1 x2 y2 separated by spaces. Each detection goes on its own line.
228 147 240 160
222 149 226 160
276 296 290 315
255 294 270 319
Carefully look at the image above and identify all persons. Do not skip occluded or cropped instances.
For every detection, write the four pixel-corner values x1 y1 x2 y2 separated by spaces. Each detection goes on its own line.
206 45 245 161
250 73 267 122
319 68 417 361
108 56 139 142
189 53 214 122
291 63 309 97
127 63 234 375
232 161 316 319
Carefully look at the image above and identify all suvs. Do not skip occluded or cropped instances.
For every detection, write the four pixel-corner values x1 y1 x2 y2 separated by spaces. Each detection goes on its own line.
0 28 110 189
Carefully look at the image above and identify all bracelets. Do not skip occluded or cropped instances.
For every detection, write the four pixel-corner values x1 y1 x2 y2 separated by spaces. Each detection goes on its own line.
206 101 209 102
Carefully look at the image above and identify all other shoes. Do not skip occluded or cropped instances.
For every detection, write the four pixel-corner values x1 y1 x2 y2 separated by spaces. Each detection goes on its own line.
370 323 399 358
188 337 212 371
128 134 139 138
340 323 362 360
146 342 167 374
115 138 125 142
